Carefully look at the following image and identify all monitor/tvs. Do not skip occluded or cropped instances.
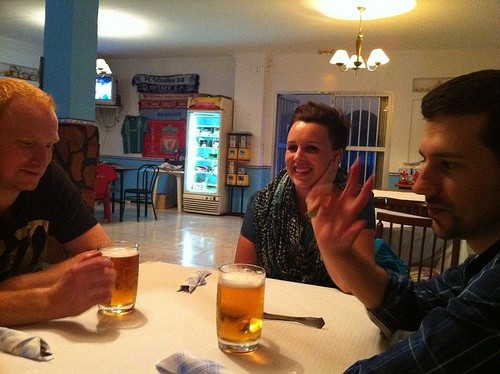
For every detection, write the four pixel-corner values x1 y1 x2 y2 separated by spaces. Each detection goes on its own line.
95 74 117 105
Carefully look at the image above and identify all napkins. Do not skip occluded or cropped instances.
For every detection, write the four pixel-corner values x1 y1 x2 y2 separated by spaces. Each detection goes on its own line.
155 353 227 374
0 326 53 361
179 270 211 294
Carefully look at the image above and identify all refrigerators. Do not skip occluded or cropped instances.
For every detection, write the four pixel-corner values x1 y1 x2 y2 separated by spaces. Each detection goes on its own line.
181 94 235 215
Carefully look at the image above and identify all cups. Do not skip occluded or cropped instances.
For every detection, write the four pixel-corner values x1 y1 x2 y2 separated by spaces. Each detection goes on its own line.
216 262 266 355
96 240 139 317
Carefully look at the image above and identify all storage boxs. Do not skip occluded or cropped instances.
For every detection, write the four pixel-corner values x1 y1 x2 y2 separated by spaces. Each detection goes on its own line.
155 193 171 209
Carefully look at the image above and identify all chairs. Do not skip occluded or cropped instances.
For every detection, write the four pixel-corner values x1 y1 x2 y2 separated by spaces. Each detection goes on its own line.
373 197 461 282
95 163 118 223
119 164 159 222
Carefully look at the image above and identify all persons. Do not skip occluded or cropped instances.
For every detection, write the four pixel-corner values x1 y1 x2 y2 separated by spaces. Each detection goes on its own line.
234 103 376 290
308 68 500 373
0 77 116 322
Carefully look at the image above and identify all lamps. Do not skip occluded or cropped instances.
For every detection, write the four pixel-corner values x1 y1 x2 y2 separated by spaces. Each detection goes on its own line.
330 7 389 76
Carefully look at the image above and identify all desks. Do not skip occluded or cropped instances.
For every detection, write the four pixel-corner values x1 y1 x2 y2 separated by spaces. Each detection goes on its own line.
0 261 402 374
151 168 184 213
112 166 140 214
373 189 469 275
